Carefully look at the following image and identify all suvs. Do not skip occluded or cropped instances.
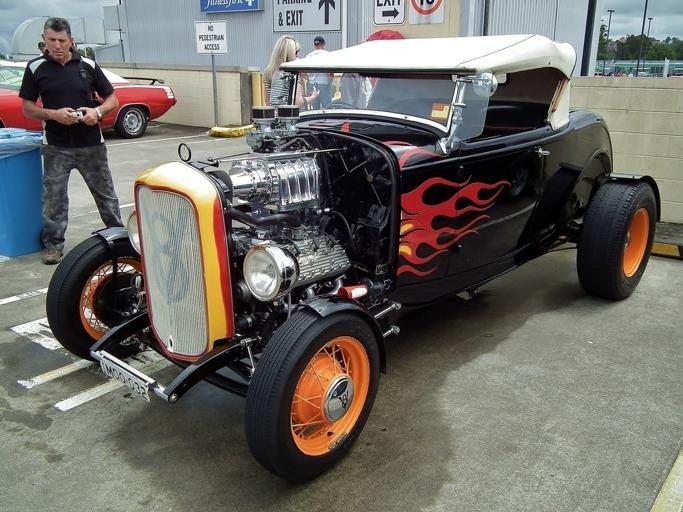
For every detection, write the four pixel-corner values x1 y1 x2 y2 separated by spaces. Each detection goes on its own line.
44 31 662 481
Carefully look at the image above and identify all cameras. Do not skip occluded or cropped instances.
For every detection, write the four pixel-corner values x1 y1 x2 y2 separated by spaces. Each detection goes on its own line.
68 111 84 119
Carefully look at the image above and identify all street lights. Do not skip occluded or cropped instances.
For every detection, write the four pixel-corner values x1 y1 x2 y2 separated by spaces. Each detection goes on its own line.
600 9 615 75
643 17 654 71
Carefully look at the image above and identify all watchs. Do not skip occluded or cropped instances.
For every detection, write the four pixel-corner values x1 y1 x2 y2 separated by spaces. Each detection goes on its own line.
95 106 102 120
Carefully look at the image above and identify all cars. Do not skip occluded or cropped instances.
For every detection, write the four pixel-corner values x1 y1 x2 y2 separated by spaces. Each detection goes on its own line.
595 71 683 79
0 58 175 138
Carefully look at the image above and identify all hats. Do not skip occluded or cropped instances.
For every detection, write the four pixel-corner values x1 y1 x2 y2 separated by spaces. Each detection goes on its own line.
314 36 325 46
38 41 46 49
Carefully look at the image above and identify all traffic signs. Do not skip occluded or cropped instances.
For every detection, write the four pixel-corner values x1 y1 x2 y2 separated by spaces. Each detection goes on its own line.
410 0 444 25
372 0 404 25
199 0 258 12
272 0 340 32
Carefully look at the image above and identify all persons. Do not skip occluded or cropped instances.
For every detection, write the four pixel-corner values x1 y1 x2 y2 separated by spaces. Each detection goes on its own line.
339 72 373 108
262 35 320 109
37 41 49 57
300 36 334 110
19 16 125 265
594 71 633 77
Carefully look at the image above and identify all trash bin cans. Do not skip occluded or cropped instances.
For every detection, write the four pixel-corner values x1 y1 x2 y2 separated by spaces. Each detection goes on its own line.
0 128 44 257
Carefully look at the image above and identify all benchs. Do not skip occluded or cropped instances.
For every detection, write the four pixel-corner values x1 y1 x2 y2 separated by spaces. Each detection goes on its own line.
382 98 549 142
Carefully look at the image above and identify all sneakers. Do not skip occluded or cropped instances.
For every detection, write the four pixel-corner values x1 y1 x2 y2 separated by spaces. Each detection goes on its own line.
42 248 64 265
